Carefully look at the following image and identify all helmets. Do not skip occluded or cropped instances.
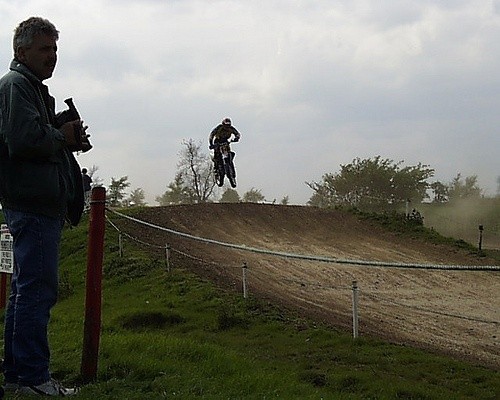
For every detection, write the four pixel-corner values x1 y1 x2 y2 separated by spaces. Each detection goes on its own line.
222 119 231 126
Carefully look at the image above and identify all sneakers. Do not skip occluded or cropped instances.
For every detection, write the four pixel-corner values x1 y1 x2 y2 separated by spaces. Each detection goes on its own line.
6 378 81 396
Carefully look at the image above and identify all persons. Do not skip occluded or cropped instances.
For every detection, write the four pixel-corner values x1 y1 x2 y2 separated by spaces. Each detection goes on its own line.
0 16 90 396
208 117 240 174
81 168 92 211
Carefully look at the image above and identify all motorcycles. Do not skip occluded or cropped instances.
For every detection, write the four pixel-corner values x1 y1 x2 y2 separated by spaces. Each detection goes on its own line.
209 138 238 189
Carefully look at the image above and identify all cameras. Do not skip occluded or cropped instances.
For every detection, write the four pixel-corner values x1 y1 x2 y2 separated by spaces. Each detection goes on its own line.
54 98 92 152
